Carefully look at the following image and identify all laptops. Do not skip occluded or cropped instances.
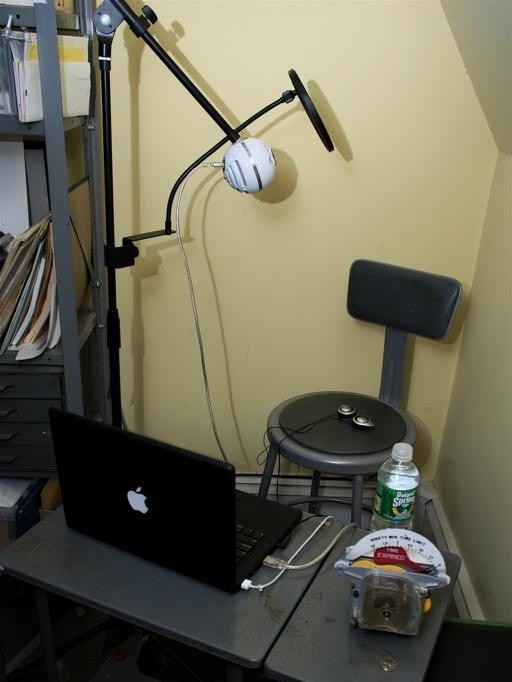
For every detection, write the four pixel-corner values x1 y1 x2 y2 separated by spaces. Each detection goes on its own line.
48 406 301 593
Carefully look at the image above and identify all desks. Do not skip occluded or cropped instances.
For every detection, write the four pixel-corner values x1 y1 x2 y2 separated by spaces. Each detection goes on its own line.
1 493 346 680
256 521 463 682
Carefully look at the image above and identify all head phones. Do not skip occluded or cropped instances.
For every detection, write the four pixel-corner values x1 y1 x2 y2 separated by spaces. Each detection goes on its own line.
338 403 376 429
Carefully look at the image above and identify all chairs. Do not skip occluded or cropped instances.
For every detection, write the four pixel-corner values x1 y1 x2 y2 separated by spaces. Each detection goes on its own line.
252 255 464 533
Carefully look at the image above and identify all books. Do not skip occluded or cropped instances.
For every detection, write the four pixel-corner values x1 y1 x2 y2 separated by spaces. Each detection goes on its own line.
1 28 96 362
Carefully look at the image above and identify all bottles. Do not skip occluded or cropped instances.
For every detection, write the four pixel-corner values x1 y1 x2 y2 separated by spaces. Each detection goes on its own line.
369 441 420 533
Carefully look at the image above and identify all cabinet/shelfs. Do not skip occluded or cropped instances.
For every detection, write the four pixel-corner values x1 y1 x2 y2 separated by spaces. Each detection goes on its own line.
0 0 126 621
